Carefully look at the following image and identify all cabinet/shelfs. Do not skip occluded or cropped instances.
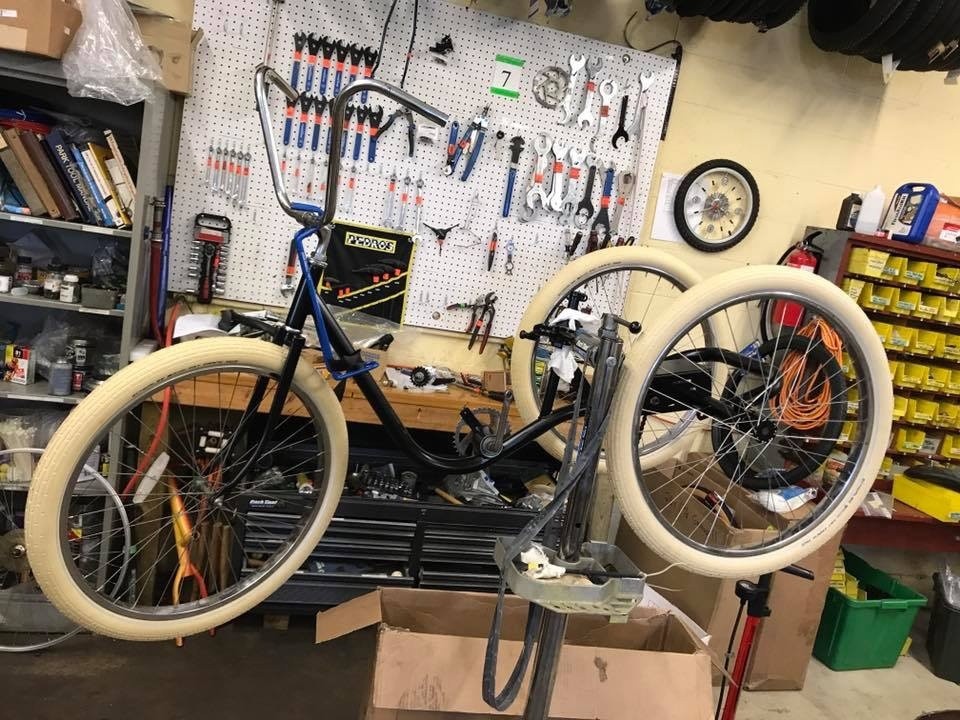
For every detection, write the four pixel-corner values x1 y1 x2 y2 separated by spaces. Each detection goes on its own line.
0 47 183 636
802 225 960 495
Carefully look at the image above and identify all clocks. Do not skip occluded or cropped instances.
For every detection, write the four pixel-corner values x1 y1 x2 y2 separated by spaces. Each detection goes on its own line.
671 158 762 256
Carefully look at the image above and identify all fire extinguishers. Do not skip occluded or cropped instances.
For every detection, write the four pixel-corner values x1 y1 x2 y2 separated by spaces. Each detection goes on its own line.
760 231 824 357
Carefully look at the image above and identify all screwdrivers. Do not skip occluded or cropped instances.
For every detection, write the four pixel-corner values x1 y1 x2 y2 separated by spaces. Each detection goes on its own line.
566 230 583 264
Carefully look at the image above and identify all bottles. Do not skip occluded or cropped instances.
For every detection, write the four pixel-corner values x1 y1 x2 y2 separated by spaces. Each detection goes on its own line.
855 185 886 236
836 191 862 231
0 255 80 304
47 338 90 395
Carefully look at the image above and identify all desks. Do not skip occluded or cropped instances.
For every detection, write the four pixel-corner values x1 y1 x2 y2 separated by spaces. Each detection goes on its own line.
131 347 592 609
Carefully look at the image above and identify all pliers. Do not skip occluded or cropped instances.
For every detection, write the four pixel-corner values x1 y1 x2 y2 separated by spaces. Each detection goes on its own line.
449 103 492 181
373 106 416 157
447 294 486 334
468 292 498 354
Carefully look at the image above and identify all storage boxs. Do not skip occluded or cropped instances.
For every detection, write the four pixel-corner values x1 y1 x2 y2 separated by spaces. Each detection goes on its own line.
313 585 740 720
0 0 205 98
230 442 567 607
920 193 960 253
614 450 960 695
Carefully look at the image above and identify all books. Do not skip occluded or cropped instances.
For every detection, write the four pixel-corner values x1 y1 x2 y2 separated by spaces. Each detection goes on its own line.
0 123 138 231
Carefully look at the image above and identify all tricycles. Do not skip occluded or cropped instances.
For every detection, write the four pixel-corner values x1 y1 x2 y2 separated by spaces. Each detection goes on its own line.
22 66 895 643
